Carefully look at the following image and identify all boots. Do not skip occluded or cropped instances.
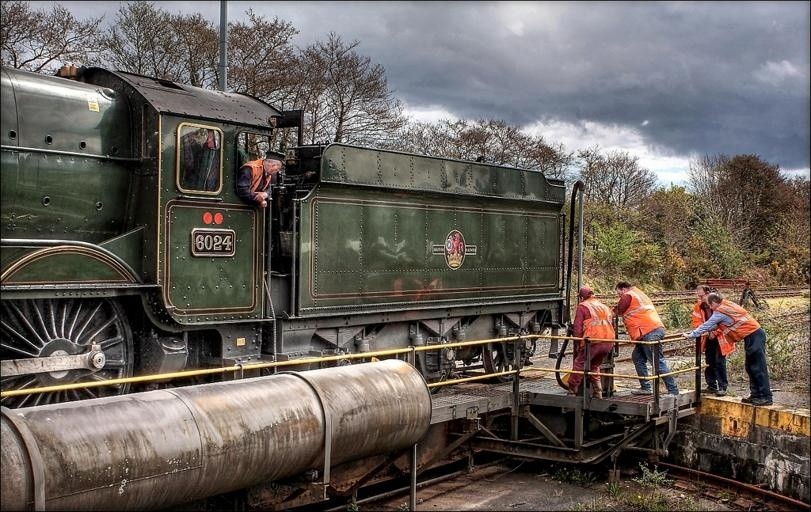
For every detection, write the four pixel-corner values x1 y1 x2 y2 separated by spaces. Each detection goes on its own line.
567 382 579 397
590 380 605 400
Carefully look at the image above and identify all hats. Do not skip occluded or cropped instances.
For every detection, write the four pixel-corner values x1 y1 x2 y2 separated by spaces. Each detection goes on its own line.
265 148 289 169
575 285 595 300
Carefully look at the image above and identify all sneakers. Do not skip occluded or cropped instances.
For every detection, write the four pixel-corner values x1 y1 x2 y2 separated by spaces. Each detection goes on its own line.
660 386 680 395
715 385 728 396
751 395 774 406
630 387 654 395
741 393 759 403
701 384 718 394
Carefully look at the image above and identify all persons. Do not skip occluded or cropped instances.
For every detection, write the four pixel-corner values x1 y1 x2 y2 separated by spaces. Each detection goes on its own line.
236 150 286 208
691 284 737 396
610 281 679 396
565 287 616 399
681 292 773 406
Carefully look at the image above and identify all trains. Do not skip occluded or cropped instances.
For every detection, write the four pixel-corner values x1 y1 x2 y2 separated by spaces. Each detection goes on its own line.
0 60 595 419
